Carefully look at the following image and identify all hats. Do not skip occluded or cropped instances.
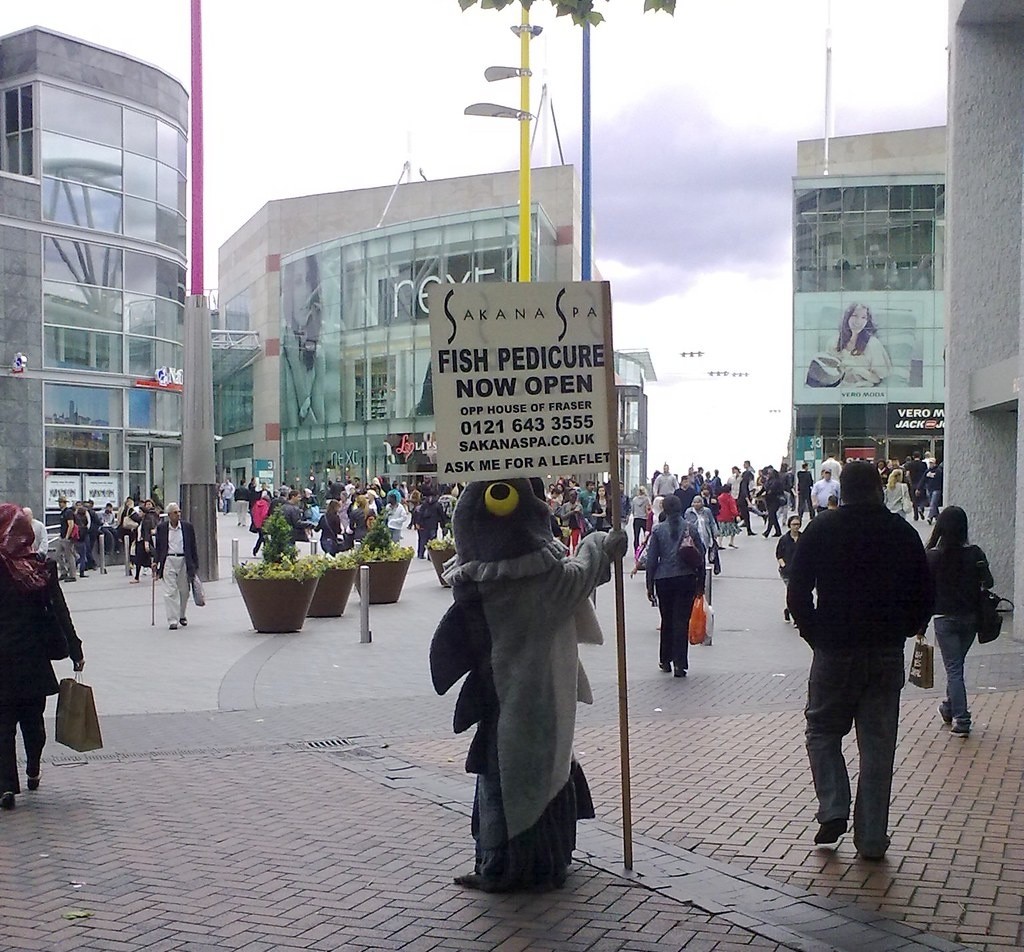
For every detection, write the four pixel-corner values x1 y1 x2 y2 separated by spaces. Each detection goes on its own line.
106 502 113 508
839 461 885 506
55 497 69 502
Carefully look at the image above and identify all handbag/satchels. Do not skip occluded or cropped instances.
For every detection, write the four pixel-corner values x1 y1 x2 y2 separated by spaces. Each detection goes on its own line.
192 574 204 606
331 538 348 551
908 639 934 689
689 593 714 646
978 590 1014 643
123 507 138 530
679 522 703 568
56 670 103 752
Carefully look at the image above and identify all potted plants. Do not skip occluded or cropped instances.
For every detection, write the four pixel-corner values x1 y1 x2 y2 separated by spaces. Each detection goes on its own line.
424 497 456 587
344 504 414 603
230 501 325 633
299 552 357 618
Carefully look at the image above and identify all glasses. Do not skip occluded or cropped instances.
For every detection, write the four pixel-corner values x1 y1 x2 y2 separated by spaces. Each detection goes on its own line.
170 510 182 514
791 523 800 526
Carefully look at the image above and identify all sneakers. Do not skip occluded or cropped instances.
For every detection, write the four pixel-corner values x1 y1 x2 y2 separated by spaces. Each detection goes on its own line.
937 704 951 724
950 729 969 737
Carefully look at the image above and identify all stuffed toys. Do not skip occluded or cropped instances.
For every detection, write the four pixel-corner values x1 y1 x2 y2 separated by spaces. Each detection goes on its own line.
428 471 628 893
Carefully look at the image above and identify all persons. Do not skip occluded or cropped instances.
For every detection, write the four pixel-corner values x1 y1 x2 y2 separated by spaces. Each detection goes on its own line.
925 505 995 737
645 495 706 677
151 503 200 629
0 503 86 809
22 507 48 561
280 253 341 429
54 495 165 584
233 464 632 563
632 452 942 554
807 302 893 388
787 460 939 860
632 511 667 607
220 479 235 515
775 515 802 627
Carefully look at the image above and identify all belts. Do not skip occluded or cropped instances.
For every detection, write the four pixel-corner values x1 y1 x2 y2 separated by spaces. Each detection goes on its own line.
167 553 185 557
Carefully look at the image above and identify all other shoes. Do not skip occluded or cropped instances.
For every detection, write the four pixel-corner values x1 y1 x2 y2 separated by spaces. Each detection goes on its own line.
169 618 187 629
784 609 798 628
718 543 739 550
130 578 139 584
658 663 686 677
814 818 847 844
59 573 76 582
860 853 885 860
28 766 42 790
0 793 14 808
